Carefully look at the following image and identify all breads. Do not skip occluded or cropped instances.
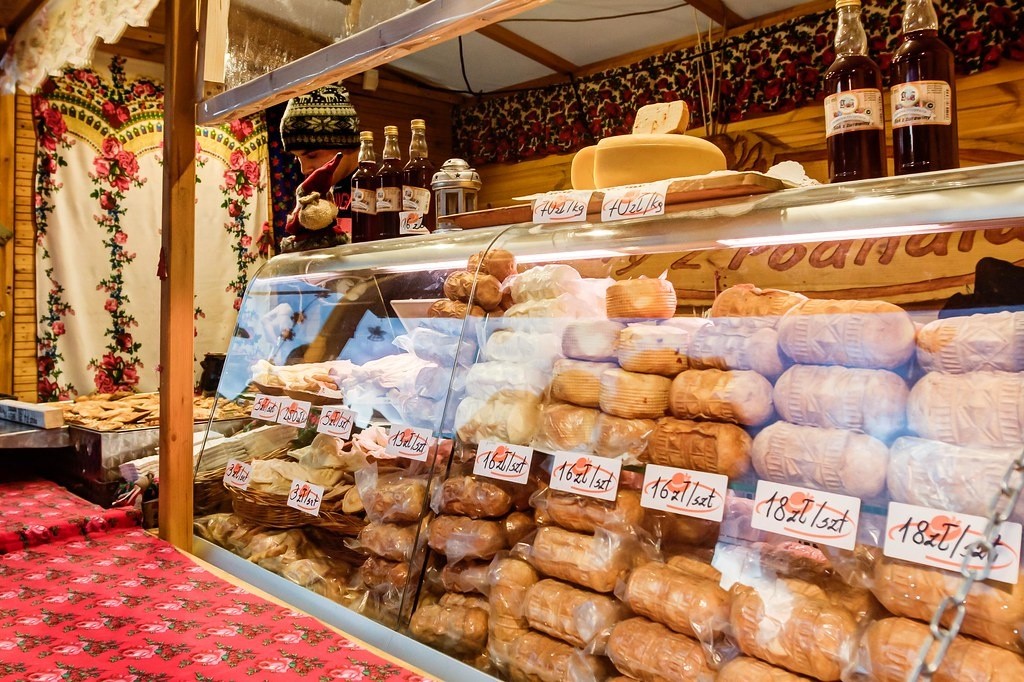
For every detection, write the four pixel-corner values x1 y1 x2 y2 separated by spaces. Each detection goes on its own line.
203 246 1024 682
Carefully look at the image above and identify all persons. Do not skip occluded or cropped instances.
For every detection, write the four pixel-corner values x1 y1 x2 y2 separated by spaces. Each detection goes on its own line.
280 77 365 237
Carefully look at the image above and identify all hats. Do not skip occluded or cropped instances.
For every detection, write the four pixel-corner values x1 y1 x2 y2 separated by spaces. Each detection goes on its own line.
280 82 362 151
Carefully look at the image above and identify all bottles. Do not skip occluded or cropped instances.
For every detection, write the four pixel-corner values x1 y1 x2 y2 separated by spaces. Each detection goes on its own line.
400 119 435 237
374 125 404 240
891 0 961 177
824 0 888 184
350 130 379 243
